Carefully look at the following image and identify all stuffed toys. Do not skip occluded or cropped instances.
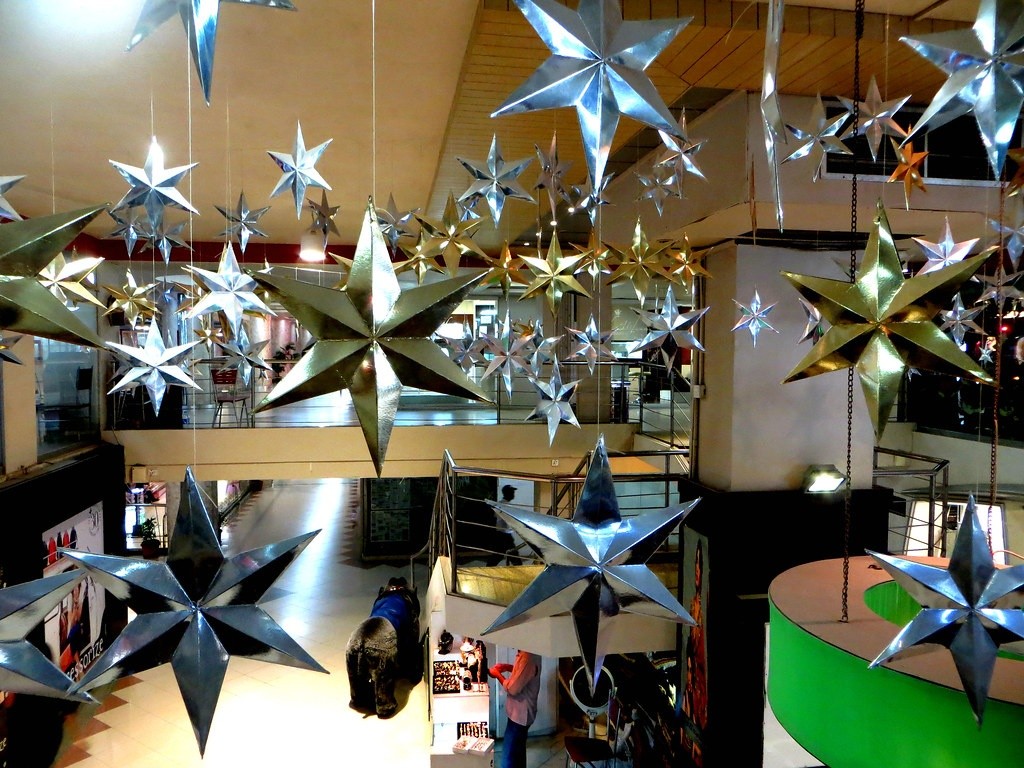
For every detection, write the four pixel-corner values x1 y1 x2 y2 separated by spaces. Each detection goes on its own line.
346 577 421 718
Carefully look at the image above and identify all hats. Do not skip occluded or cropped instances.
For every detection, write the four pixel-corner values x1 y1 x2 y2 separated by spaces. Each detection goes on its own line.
502 485 517 494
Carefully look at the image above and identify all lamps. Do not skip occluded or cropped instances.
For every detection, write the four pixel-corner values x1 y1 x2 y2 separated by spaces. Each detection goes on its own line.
802 464 847 494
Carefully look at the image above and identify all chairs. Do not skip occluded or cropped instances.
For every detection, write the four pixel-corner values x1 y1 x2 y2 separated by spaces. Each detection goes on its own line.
37 365 94 441
211 368 250 428
564 689 622 768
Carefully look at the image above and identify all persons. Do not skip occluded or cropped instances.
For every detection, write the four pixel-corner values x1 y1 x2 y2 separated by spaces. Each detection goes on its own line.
489 648 542 768
485 484 523 567
